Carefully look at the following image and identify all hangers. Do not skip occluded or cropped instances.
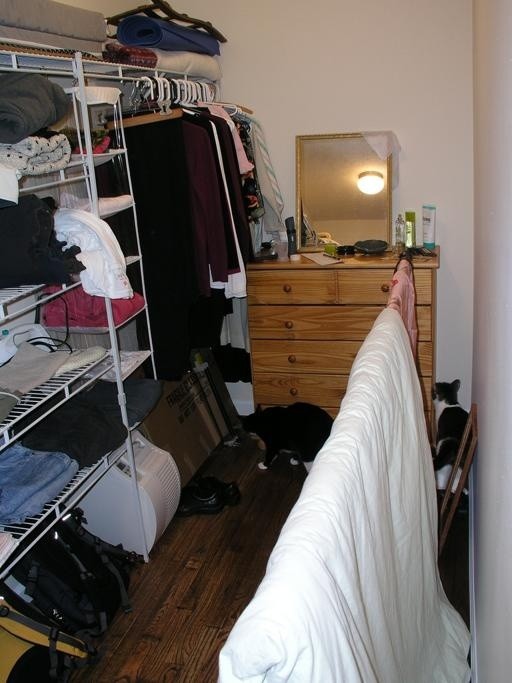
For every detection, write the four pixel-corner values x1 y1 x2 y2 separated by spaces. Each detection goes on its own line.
96 76 256 130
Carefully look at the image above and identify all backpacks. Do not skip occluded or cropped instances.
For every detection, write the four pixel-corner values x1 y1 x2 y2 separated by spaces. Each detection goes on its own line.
1 499 138 654
1 597 90 682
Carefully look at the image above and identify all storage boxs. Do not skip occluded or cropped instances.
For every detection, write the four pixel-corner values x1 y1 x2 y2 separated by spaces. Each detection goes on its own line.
136 346 241 487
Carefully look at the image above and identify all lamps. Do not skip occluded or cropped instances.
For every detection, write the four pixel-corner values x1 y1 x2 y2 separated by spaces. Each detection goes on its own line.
355 167 387 201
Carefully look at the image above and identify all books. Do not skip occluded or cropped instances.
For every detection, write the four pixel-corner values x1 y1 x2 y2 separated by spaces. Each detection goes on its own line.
301 252 344 266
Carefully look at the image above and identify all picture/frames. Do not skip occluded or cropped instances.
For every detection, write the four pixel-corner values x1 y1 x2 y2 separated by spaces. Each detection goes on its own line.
438 400 478 559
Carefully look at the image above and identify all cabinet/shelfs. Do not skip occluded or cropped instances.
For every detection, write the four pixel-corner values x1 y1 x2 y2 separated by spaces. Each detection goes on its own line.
0 41 219 583
244 244 441 456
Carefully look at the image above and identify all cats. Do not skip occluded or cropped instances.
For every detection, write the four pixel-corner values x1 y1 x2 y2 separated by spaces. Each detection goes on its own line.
431 379 473 496
235 402 335 471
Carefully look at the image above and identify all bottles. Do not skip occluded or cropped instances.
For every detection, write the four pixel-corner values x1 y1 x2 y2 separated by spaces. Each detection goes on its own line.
396 215 405 253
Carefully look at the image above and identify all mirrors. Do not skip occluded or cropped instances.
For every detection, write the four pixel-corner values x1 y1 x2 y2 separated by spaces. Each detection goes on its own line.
295 130 393 252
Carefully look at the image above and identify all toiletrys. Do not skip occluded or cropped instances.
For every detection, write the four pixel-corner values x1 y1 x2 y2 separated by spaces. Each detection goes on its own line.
325 245 335 254
290 253 302 262
285 215 297 256
404 211 418 248
422 203 436 251
394 211 406 250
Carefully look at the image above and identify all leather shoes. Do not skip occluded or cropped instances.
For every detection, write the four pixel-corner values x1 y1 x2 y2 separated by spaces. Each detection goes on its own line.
175 487 224 517
189 475 242 506
